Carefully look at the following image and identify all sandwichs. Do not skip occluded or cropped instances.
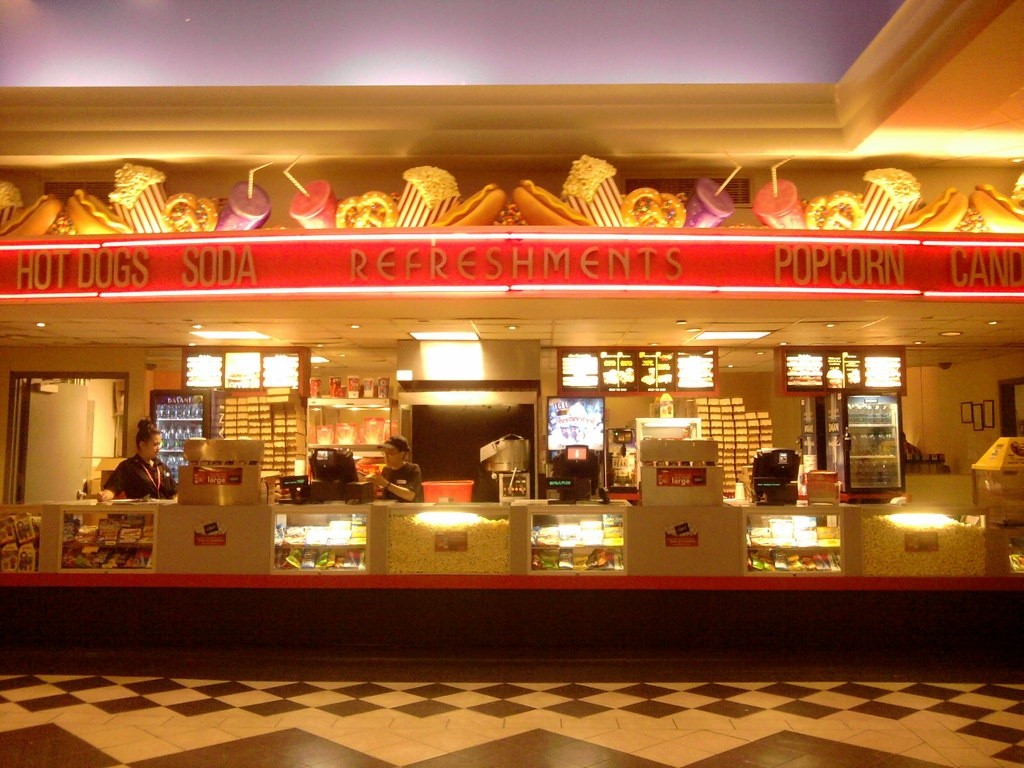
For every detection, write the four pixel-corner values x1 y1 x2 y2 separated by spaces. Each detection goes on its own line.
893 189 968 232
967 184 1024 234
0 192 61 237
426 182 505 226
511 180 597 227
67 190 134 236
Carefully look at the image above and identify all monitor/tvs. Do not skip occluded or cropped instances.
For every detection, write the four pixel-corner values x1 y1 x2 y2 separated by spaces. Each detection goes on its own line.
498 474 530 503
310 447 360 483
753 447 800 482
565 445 589 463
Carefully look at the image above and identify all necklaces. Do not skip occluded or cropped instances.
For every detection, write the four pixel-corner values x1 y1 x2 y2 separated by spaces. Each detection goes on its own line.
140 463 165 499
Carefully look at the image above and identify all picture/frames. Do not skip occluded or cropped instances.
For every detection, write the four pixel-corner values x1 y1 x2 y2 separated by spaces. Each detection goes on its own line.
961 400 995 431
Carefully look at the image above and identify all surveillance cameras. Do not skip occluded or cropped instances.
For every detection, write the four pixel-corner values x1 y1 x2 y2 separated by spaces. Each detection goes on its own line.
938 362 952 369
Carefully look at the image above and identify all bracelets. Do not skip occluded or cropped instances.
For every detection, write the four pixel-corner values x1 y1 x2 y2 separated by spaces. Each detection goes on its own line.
385 481 391 491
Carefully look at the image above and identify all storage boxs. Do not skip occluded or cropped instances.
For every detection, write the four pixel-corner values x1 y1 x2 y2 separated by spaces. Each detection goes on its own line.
687 396 773 502
224 387 307 479
94 458 129 499
479 432 530 471
421 479 475 502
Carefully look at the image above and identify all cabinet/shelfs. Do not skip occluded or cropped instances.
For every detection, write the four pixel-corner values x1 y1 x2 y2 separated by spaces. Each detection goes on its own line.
305 397 400 483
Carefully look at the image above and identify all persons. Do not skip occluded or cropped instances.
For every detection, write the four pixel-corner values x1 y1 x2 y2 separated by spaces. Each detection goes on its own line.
365 434 424 502
903 432 921 454
97 415 179 502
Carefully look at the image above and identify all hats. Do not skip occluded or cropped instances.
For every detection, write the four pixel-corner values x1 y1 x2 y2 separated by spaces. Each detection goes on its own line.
377 435 408 452
